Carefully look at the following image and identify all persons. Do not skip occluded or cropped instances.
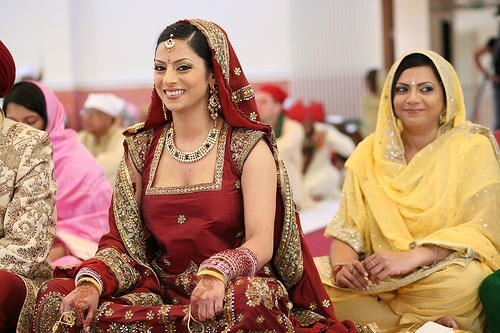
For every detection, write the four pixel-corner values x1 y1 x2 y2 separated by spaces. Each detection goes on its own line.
35 18 359 332
475 33 500 138
64 84 378 218
310 50 500 332
0 38 57 333
3 79 114 269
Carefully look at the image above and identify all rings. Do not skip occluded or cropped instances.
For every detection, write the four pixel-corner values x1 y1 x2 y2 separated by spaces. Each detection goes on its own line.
350 267 355 273
52 310 77 333
371 260 376 266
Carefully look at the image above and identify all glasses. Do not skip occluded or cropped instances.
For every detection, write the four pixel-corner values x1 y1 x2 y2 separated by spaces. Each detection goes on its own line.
80 109 102 117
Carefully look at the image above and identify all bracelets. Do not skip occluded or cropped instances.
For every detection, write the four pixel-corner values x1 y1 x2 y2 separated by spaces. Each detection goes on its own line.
420 243 440 269
74 267 104 295
197 246 258 286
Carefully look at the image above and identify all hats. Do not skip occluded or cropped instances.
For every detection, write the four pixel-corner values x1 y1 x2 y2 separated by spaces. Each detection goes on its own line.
84 92 124 118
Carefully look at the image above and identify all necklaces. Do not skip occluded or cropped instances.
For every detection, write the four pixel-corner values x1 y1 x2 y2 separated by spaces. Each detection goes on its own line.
166 122 219 163
